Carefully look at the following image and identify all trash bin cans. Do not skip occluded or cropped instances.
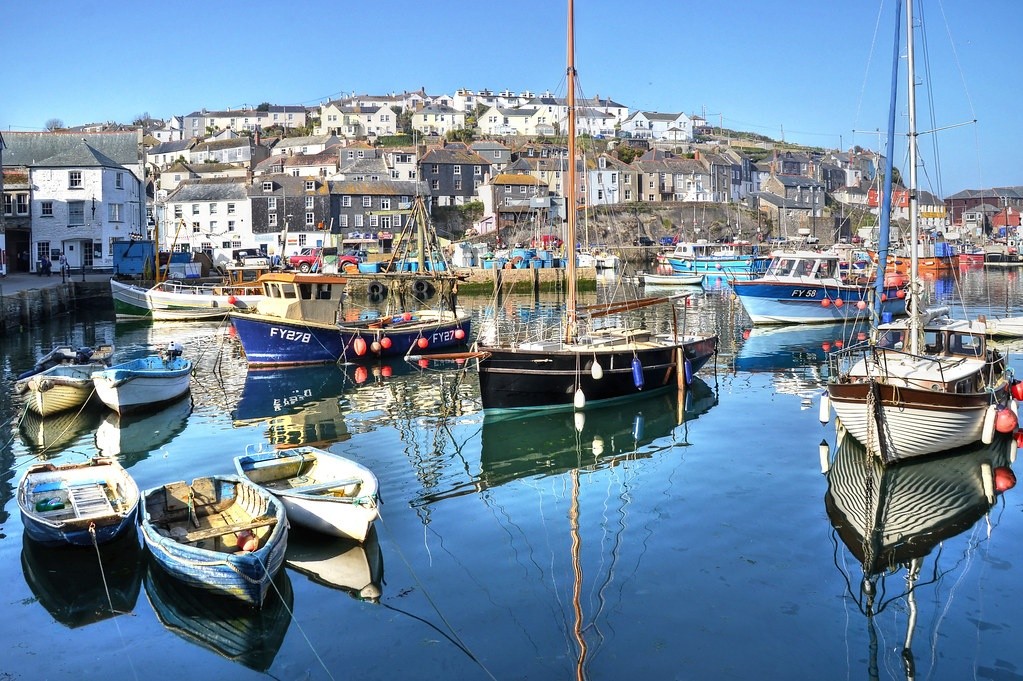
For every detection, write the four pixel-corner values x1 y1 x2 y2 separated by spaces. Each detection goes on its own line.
358 262 381 273
512 248 580 269
484 260 503 269
399 260 448 272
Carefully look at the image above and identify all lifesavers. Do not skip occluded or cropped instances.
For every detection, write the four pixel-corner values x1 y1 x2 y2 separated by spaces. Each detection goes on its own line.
368 281 384 294
413 292 429 301
412 279 429 293
368 294 384 303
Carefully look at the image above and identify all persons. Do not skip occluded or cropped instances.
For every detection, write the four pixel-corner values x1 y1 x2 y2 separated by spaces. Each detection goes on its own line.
41 256 51 276
22 251 30 272
59 252 67 275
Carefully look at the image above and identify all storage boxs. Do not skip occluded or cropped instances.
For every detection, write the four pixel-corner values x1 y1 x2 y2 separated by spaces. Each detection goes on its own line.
36 497 65 512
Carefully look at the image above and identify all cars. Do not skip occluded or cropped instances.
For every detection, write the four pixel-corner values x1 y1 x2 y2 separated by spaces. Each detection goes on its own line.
525 219 863 250
227 246 373 269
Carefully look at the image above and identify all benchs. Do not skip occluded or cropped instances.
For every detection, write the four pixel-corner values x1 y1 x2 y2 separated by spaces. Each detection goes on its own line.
243 454 317 472
32 477 118 492
282 477 361 493
170 518 277 544
36 497 124 517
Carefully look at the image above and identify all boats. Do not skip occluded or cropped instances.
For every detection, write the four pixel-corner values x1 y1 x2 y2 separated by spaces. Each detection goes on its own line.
19 523 388 681
94 388 196 462
17 399 102 457
14 343 115 416
231 360 467 428
233 440 382 543
728 324 900 375
109 274 472 378
406 376 720 681
136 470 291 610
91 342 194 417
821 427 1023 681
655 239 916 326
15 454 141 549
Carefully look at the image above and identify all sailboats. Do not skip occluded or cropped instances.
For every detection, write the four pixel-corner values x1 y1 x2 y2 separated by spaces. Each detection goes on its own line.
474 0 719 417
821 0 1017 469
